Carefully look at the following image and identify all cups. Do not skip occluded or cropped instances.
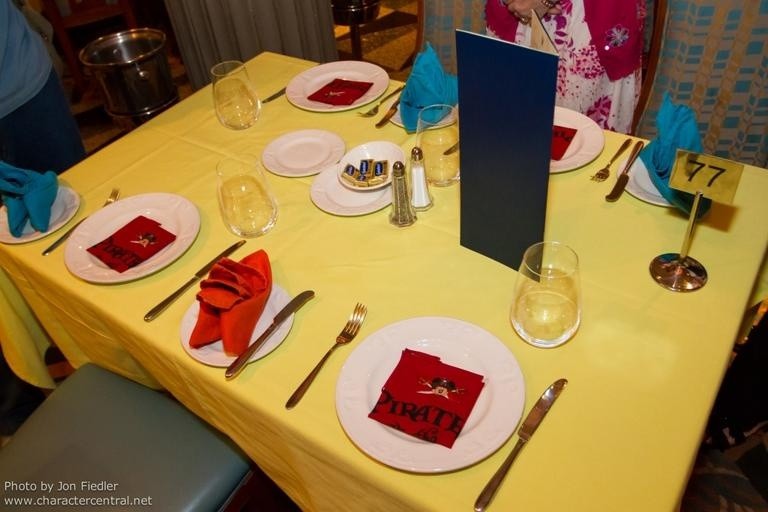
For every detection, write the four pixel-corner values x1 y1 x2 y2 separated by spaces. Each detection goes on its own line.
415 103 461 187
509 241 581 349
215 152 278 238
210 60 261 131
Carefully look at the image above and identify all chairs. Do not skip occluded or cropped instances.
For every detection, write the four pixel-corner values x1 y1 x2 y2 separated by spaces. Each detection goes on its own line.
1 360 265 512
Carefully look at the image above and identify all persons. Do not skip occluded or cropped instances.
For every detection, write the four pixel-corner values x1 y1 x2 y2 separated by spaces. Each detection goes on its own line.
485 1 647 134
1 0 85 175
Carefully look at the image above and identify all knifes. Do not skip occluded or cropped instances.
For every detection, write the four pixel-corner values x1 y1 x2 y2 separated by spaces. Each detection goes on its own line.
143 240 247 321
261 86 286 104
225 290 315 378
606 141 649 205
474 380 567 512
375 95 402 127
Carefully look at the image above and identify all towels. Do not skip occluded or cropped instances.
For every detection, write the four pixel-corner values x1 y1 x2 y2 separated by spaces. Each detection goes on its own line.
551 124 579 163
86 214 177 275
306 77 375 108
368 347 488 451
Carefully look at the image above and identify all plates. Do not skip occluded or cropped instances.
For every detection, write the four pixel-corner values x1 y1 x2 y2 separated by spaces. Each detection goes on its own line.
335 316 525 475
64 193 201 285
262 129 407 217
548 106 605 174
0 186 80 245
285 61 389 113
620 154 677 214
180 283 295 367
385 96 458 129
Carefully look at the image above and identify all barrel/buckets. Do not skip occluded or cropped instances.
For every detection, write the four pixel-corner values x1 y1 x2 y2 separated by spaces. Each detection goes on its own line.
79 27 172 113
104 85 181 133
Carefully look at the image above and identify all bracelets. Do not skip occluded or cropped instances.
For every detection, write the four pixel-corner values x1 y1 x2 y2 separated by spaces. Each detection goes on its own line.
541 0 557 8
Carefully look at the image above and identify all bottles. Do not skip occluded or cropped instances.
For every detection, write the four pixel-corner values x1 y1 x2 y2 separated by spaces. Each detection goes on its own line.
389 161 417 228
409 147 433 211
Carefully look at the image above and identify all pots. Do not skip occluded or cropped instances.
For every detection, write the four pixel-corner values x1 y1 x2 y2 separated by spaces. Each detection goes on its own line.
77 27 180 130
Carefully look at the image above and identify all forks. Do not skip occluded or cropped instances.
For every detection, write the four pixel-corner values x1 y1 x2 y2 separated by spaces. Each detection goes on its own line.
356 86 405 117
285 302 369 411
41 188 121 256
588 138 635 183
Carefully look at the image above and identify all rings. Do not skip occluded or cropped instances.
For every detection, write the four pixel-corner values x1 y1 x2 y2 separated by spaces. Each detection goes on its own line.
521 17 528 23
